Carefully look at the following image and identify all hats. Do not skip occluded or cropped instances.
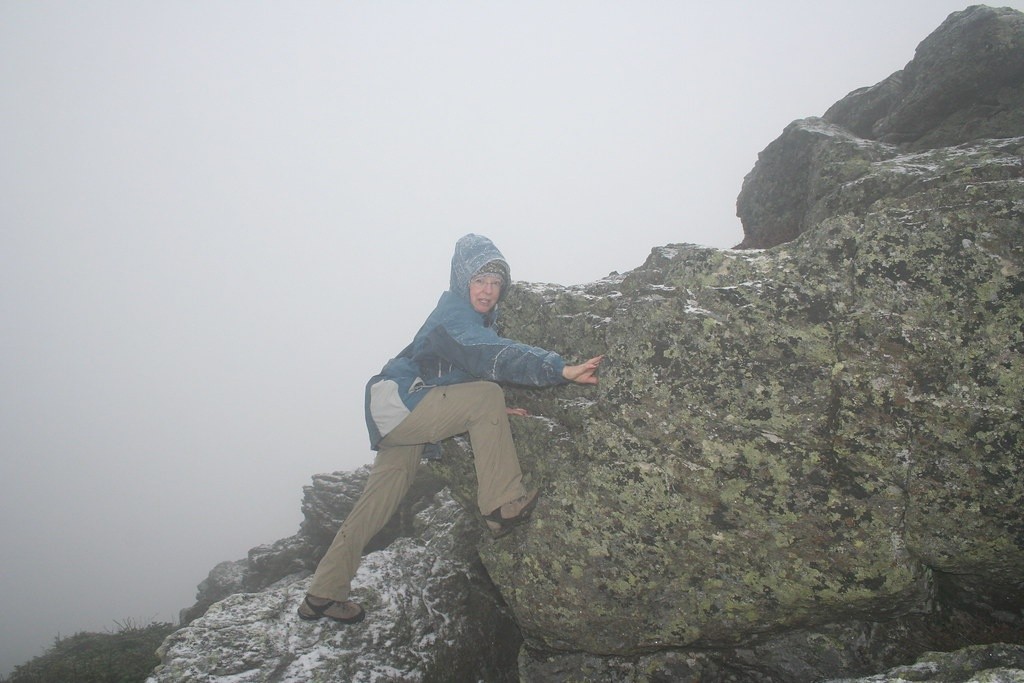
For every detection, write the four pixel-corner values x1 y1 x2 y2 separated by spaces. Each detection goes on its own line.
472 262 507 285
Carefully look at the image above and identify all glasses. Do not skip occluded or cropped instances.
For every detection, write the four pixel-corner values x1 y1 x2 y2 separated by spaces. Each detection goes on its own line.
474 277 500 287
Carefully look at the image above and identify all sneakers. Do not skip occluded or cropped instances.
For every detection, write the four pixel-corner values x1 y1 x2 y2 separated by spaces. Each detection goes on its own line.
298 596 365 624
485 485 540 539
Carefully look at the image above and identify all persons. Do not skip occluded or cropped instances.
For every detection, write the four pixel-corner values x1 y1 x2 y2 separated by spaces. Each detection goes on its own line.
298 233 606 623
